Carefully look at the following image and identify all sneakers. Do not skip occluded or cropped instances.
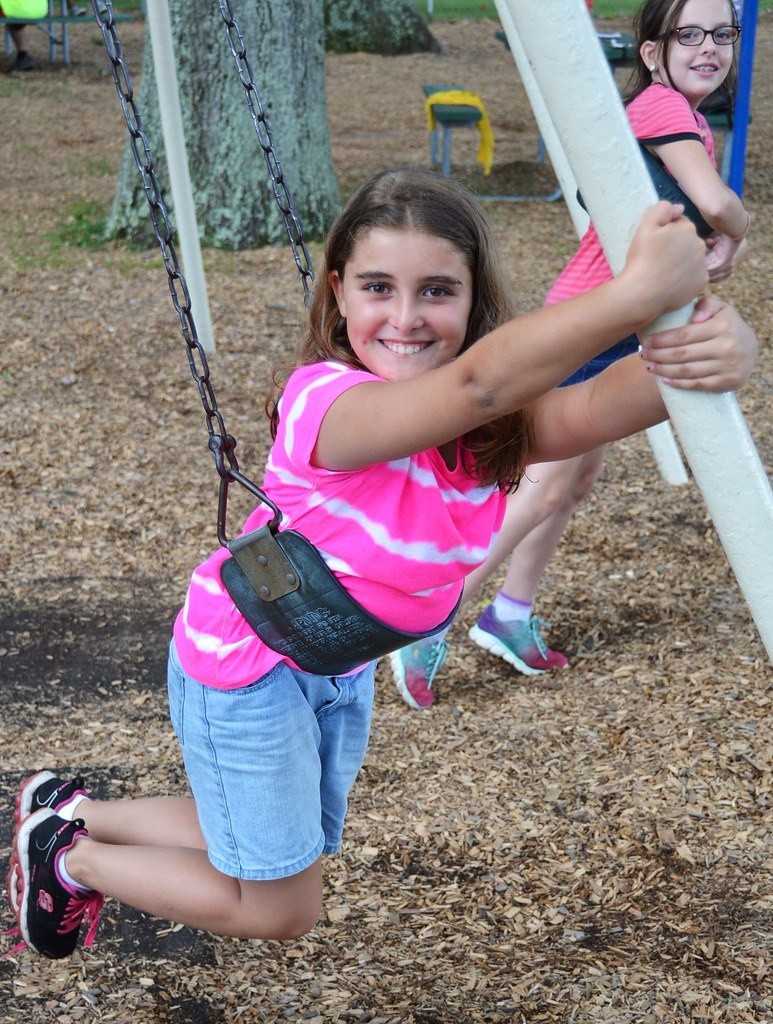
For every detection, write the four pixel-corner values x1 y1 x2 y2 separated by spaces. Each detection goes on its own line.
390 624 451 708
16 807 96 959
467 605 570 676
8 770 88 915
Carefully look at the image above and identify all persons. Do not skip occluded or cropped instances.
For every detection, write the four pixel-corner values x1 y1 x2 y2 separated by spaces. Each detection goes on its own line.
6 167 761 962
1 0 49 74
389 0 756 713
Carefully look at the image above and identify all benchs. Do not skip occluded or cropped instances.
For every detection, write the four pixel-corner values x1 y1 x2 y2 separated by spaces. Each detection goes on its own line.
423 79 481 197
0 14 136 75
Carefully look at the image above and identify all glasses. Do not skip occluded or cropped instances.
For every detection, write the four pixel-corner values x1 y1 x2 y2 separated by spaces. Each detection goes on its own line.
652 25 741 46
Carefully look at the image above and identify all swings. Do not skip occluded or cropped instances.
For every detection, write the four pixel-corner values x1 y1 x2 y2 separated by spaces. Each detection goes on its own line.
576 139 716 241
86 0 464 680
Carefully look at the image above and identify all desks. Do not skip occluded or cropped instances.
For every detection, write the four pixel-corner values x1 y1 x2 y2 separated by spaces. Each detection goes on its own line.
492 26 653 199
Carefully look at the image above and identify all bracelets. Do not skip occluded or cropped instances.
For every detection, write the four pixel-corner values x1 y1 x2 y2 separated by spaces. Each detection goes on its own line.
723 210 751 243
